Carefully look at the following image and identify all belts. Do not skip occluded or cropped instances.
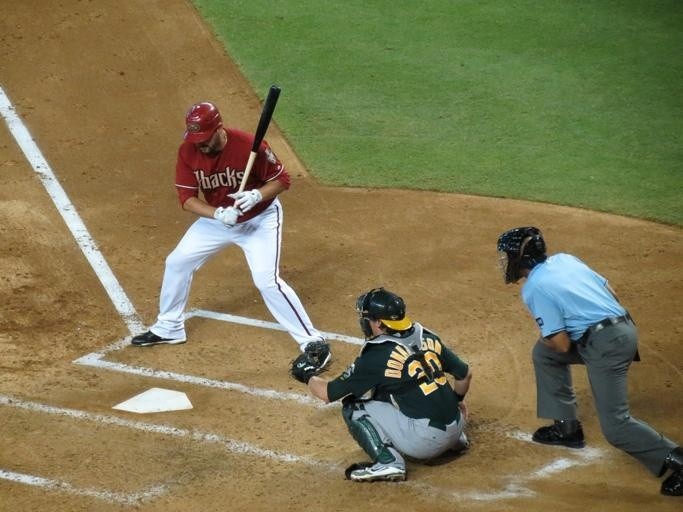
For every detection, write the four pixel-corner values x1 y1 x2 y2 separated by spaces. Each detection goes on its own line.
579 310 630 350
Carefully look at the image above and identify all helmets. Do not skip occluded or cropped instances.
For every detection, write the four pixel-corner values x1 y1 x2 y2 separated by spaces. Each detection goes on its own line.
183 99 224 144
495 225 547 286
354 286 414 341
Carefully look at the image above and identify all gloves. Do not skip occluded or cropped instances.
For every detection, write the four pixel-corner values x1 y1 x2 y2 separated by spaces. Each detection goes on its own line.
228 188 265 212
212 204 242 227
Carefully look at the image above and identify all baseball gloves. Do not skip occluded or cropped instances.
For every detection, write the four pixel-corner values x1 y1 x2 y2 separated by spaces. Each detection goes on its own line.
292 352 319 385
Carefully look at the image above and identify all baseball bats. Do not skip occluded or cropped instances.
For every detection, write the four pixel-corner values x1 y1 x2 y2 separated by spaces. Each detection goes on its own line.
234 86 280 208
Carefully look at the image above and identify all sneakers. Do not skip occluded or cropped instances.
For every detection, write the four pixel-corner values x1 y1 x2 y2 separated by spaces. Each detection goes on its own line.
659 446 683 497
448 432 471 453
131 329 189 348
288 342 333 379
533 420 585 450
346 460 407 482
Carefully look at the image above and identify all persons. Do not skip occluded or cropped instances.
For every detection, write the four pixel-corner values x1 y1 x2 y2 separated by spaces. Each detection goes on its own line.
290 285 472 482
496 225 683 496
129 102 332 372
